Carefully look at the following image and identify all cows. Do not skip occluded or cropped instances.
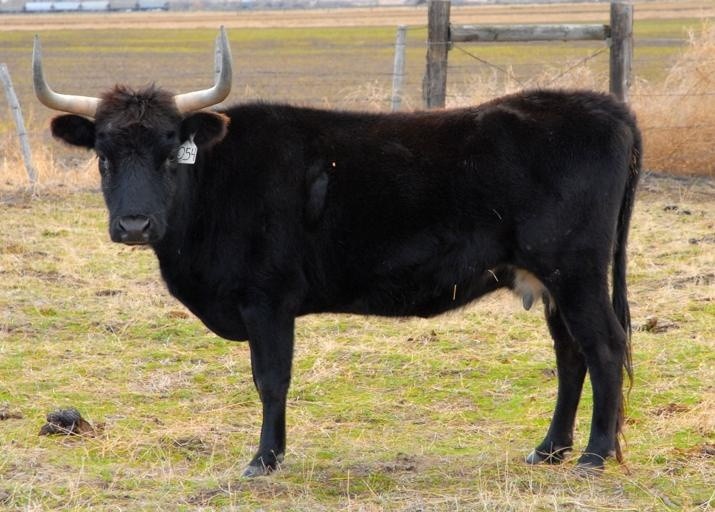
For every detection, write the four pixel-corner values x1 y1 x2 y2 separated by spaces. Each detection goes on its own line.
33 23 643 480
